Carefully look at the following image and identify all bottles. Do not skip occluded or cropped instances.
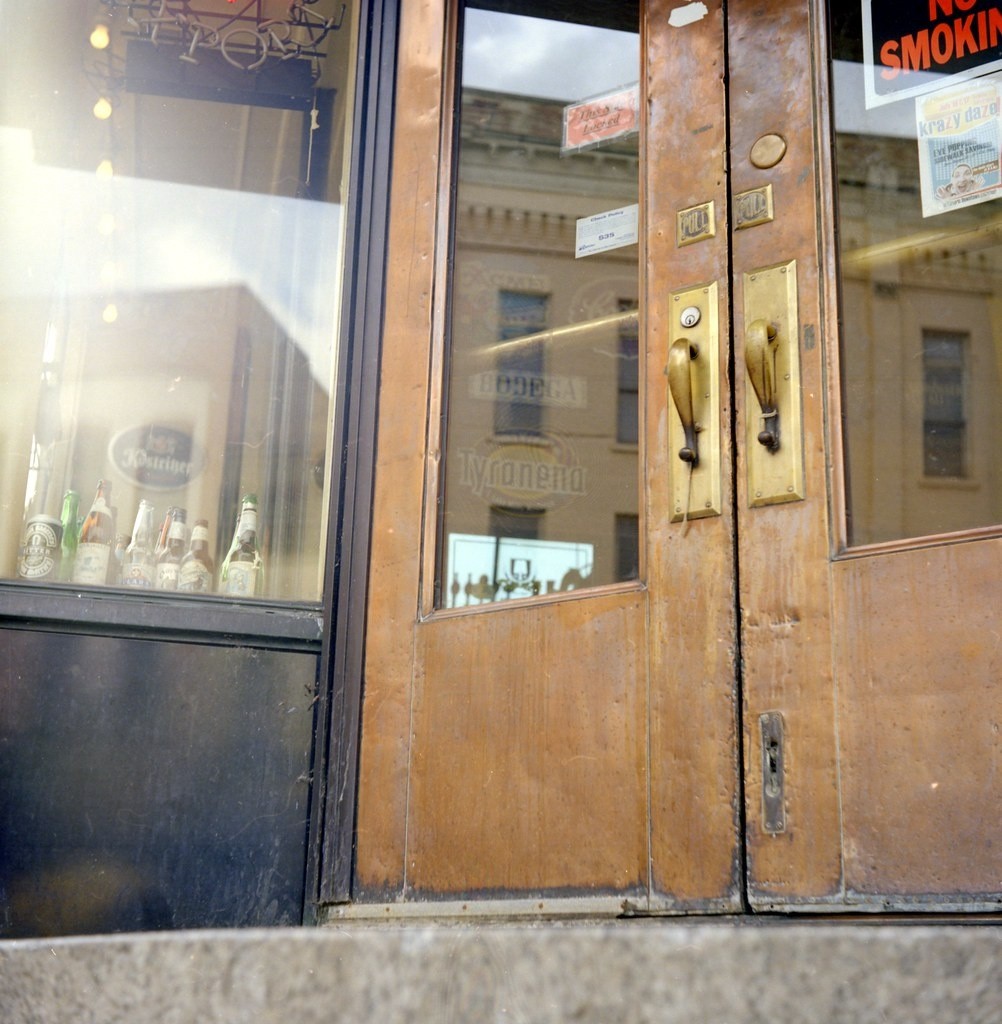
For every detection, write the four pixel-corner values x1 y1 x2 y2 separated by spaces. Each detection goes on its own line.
14 479 265 599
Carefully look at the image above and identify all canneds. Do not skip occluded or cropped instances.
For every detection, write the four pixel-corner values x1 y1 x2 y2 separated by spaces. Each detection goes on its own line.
18 514 65 581
